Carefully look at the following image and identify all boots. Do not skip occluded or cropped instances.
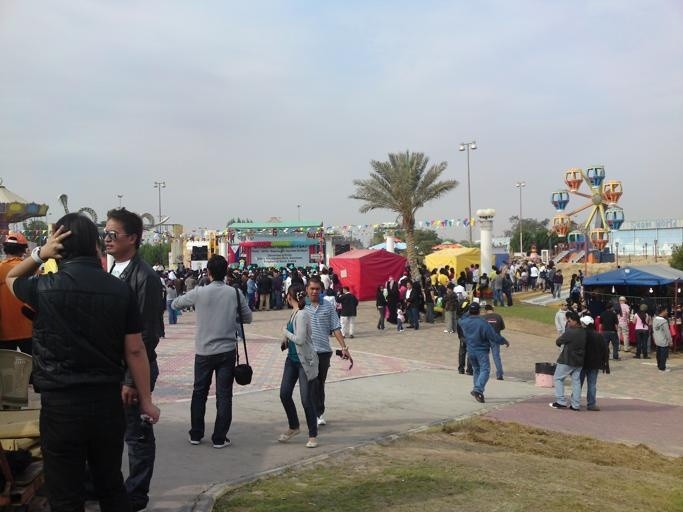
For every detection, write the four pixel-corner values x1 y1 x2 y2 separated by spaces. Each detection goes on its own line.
633 352 640 358
643 353 650 359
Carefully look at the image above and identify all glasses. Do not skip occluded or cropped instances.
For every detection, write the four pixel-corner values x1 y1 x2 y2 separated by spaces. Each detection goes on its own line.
102 230 128 240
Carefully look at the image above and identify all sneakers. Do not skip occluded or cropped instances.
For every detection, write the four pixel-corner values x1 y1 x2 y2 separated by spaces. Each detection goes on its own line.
570 405 579 410
213 438 231 448
459 370 465 374
660 369 670 373
614 358 621 361
497 376 504 379
279 429 299 441
188 429 201 444
471 390 485 403
549 402 567 408
589 405 600 411
307 436 320 448
316 413 326 425
467 371 473 375
132 502 148 512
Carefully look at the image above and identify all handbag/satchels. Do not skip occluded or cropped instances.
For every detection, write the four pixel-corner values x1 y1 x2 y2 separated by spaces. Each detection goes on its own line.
598 351 606 370
234 364 253 385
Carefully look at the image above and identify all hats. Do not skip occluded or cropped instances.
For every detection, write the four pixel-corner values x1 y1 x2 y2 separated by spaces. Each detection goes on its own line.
469 302 480 310
580 315 594 325
619 297 625 300
640 304 647 310
566 312 579 322
4 233 29 249
561 301 568 305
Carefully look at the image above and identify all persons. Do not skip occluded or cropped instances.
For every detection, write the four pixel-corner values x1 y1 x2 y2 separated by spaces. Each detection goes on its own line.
305 277 351 429
4 212 162 512
547 311 586 412
455 299 474 375
277 283 322 448
167 255 252 449
153 260 361 340
0 229 43 385
98 208 165 512
578 315 608 413
457 302 510 403
554 296 675 374
482 303 507 380
373 256 584 334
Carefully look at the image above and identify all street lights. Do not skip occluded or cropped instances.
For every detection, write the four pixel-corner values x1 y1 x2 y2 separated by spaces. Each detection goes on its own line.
476 208 496 289
118 193 123 208
153 176 165 235
515 179 525 257
458 135 477 244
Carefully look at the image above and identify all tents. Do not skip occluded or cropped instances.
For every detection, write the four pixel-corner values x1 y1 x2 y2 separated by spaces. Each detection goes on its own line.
329 249 408 302
421 246 496 294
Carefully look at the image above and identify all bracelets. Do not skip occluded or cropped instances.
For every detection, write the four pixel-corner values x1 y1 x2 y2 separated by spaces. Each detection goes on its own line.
342 346 349 351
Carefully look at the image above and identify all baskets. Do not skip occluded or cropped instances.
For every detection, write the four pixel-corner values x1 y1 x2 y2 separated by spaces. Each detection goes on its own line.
0 349 33 406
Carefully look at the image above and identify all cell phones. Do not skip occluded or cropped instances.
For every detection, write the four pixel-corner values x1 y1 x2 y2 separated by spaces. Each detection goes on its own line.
280 344 286 351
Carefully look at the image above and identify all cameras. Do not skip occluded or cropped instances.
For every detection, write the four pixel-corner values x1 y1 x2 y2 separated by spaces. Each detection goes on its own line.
336 350 348 359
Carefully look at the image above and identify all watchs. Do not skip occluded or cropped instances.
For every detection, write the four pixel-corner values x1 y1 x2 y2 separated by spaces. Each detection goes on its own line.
29 246 48 264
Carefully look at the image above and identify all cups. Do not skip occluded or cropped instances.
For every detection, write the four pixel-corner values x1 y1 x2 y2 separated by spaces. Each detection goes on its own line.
135 412 154 442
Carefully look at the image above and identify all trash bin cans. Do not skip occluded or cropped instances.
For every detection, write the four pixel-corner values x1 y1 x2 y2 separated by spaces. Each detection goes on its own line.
535 362 557 387
